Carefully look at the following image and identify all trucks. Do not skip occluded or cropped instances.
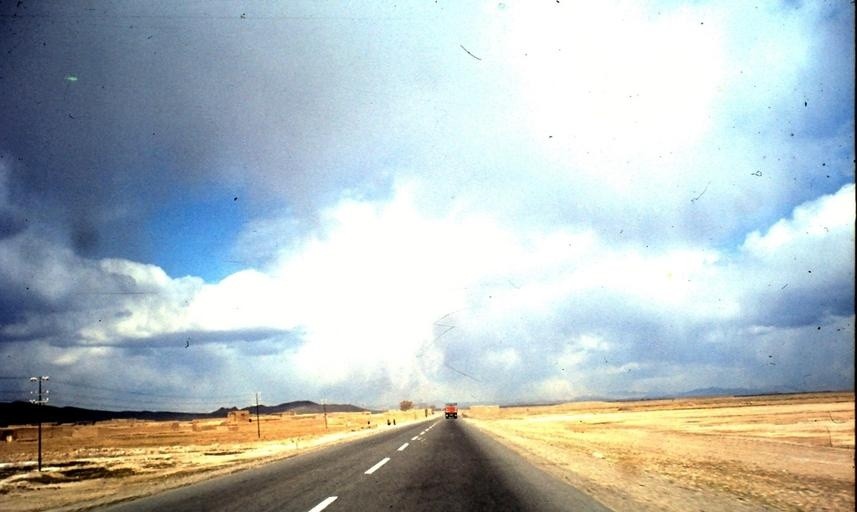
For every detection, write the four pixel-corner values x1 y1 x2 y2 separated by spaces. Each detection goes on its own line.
444 402 457 419
